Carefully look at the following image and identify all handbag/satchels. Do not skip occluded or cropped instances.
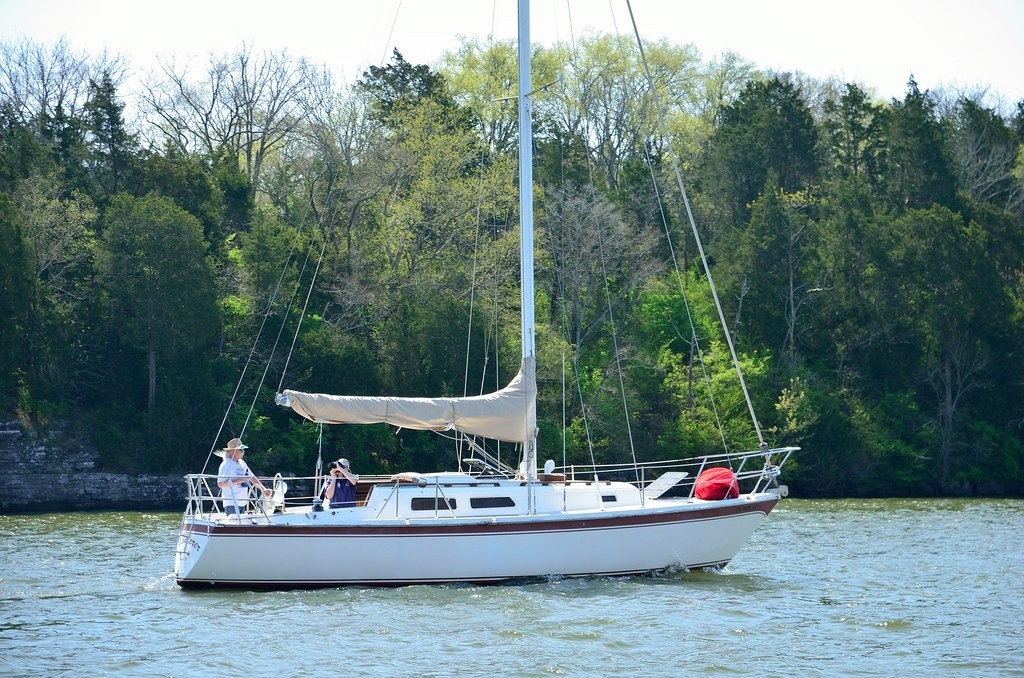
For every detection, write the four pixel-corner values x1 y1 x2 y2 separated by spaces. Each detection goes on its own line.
695 467 739 499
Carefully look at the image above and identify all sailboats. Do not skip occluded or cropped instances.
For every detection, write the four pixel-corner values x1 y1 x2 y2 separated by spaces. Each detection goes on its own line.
173 0 802 586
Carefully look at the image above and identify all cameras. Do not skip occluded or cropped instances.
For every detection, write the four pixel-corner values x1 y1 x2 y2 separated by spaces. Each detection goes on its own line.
328 463 337 469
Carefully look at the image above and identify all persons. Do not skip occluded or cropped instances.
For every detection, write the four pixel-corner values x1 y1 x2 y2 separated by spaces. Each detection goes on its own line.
217 438 271 517
323 458 358 509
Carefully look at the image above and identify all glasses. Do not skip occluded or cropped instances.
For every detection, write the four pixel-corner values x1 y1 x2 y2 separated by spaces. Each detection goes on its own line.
237 449 244 452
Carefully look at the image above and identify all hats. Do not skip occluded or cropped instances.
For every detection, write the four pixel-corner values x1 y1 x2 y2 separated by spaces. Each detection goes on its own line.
338 458 350 467
223 438 249 451
